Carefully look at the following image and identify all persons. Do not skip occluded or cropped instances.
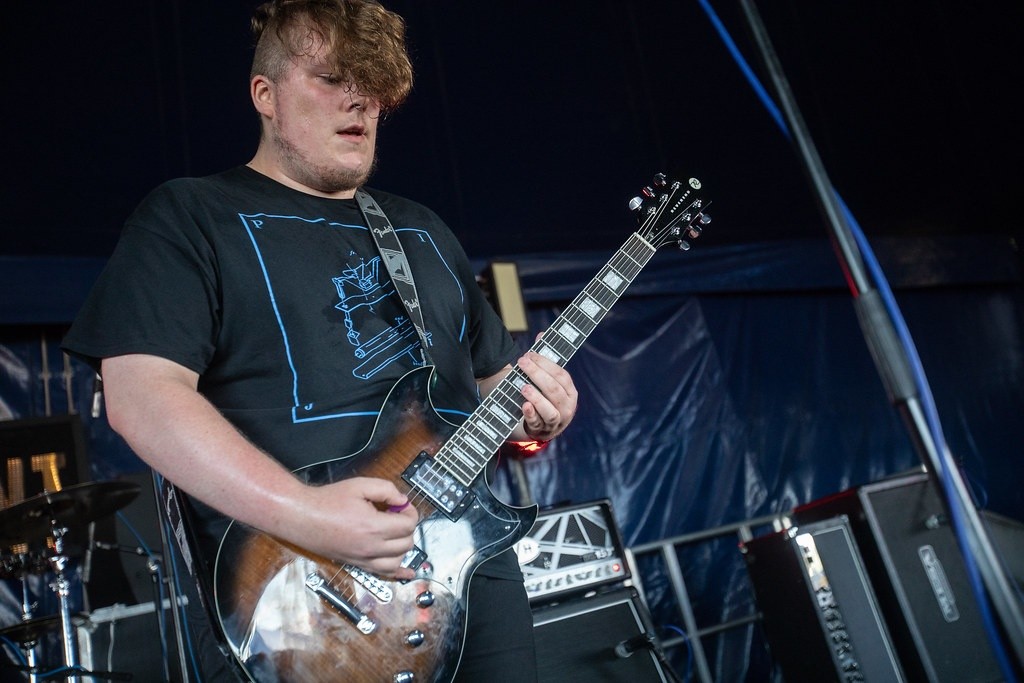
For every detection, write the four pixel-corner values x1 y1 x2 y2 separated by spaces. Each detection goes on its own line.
57 0 578 683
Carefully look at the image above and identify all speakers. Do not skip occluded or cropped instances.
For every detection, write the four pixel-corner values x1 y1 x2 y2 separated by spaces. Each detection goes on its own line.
796 472 1015 682
530 586 673 683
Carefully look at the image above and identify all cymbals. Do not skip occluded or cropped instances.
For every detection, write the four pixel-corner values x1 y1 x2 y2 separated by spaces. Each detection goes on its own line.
0 611 90 645
0 480 141 529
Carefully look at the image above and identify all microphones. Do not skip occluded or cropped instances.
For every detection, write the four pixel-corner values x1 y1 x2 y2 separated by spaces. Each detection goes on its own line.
90 374 103 418
924 512 951 530
614 632 651 659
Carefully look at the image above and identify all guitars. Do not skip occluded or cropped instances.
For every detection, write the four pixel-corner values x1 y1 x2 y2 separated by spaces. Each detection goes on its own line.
206 168 716 683
